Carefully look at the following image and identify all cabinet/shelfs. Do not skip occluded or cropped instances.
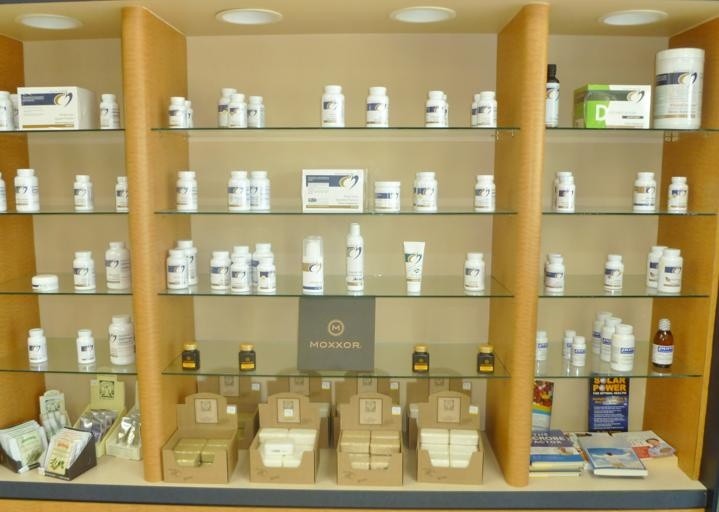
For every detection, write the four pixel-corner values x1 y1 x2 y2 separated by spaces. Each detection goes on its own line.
0 0 718 512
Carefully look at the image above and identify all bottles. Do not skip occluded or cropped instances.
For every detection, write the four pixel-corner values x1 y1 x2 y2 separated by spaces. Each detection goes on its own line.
632 170 659 213
545 63 561 127
561 329 587 369
106 240 130 293
463 251 486 293
175 168 269 214
476 343 496 373
302 237 325 294
76 329 96 365
320 86 345 127
210 243 278 292
180 342 203 371
375 180 401 214
601 253 624 291
365 85 390 127
0 89 20 132
0 167 128 213
534 330 550 362
216 86 264 129
425 91 448 127
178 240 198 285
645 243 684 293
665 174 690 213
542 253 565 293
592 311 637 371
97 91 120 130
551 170 576 213
167 249 189 289
471 90 497 127
411 345 431 373
239 344 257 371
412 167 497 212
652 318 676 369
108 314 135 366
73 250 97 290
168 96 194 128
27 327 48 372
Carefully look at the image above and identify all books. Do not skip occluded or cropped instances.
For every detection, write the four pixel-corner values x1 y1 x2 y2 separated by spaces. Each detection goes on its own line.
590 371 630 431
531 378 554 432
529 426 680 480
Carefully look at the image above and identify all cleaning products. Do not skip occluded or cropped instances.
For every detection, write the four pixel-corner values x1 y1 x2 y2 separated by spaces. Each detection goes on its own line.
301 234 324 294
